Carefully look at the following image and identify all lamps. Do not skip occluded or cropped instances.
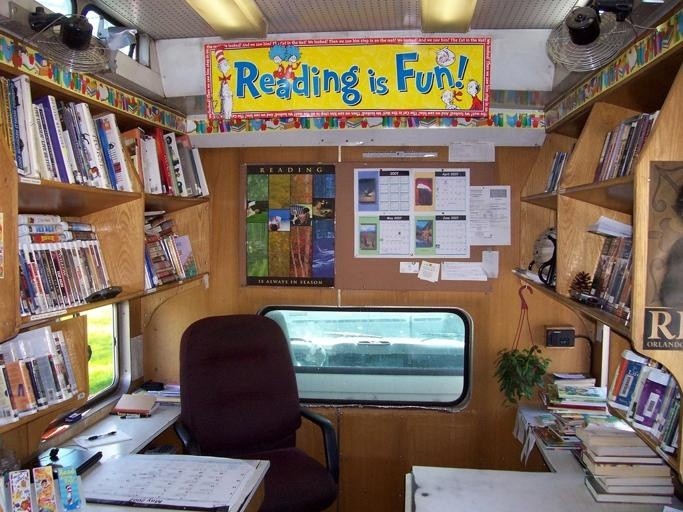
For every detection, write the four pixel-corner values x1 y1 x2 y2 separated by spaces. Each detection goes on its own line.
181 0 272 42
418 1 482 35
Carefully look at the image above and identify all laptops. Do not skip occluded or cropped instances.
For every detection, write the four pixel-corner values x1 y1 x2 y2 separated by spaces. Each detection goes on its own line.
18 446 103 483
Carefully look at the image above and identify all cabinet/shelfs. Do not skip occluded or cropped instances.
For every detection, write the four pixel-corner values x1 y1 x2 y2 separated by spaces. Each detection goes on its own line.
508 3 682 498
0 27 210 475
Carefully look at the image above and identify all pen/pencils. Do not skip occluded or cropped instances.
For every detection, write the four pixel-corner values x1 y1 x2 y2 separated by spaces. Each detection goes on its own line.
88 431 117 440
120 414 151 419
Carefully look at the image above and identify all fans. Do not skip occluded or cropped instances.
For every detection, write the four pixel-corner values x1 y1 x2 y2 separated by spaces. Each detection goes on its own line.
545 1 661 73
0 1 114 79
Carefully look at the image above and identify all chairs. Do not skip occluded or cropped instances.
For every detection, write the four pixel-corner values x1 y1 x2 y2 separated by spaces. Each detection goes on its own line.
164 308 343 511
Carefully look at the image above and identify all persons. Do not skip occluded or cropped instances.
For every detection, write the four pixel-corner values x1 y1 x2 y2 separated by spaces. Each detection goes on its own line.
269 216 282 232
292 207 311 226
247 202 262 218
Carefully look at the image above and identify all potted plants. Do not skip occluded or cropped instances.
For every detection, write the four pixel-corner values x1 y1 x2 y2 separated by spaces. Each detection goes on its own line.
493 344 553 405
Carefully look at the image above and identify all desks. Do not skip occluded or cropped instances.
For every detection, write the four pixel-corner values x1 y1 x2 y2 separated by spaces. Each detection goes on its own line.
13 391 271 511
399 394 683 510
